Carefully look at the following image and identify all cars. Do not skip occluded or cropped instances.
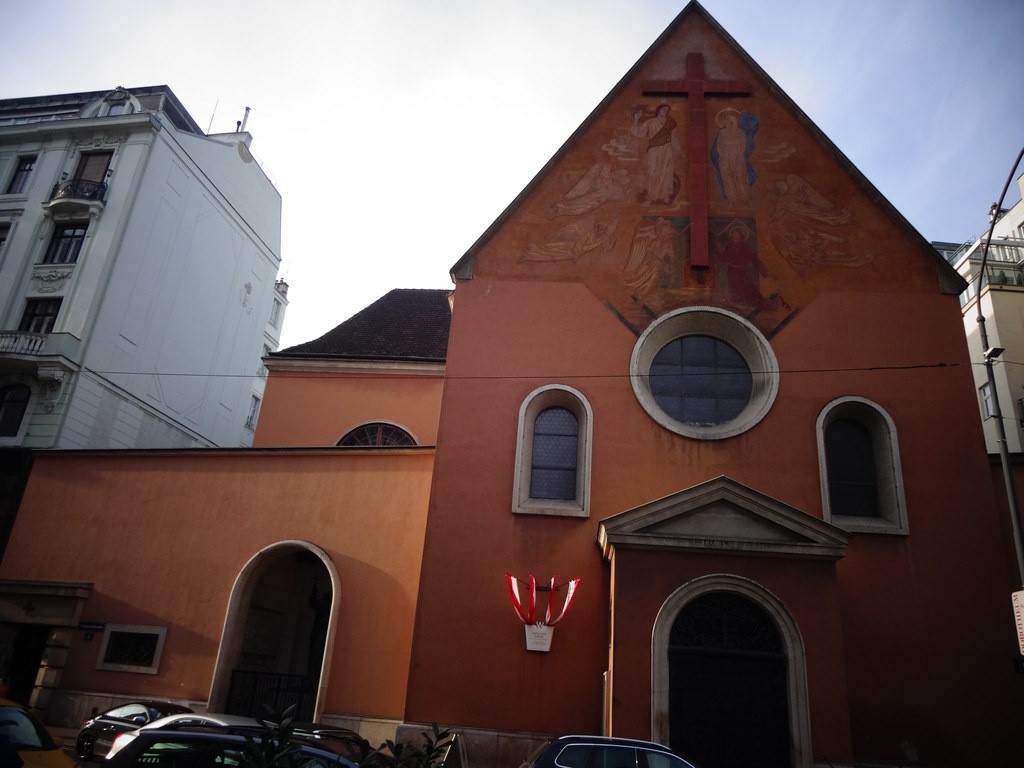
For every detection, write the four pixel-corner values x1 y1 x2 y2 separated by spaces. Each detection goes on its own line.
0 699 395 768
518 734 697 768
